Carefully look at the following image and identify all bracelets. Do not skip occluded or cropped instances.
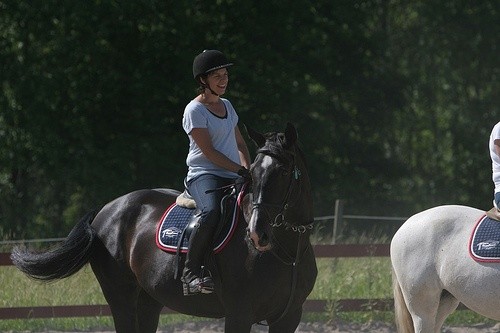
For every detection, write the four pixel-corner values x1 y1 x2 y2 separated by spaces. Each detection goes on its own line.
237 166 246 176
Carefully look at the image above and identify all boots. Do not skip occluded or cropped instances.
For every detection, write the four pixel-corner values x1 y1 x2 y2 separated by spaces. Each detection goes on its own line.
180 219 214 296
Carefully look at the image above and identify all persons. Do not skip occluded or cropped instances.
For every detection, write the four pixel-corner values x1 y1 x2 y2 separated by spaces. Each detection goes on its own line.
182 48 252 297
489 120 500 212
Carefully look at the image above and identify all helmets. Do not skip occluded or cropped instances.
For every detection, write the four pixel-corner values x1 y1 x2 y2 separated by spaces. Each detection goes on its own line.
193 50 235 80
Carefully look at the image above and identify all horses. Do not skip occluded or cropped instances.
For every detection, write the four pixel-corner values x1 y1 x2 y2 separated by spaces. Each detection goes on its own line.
390 204 500 333
10 122 318 333
